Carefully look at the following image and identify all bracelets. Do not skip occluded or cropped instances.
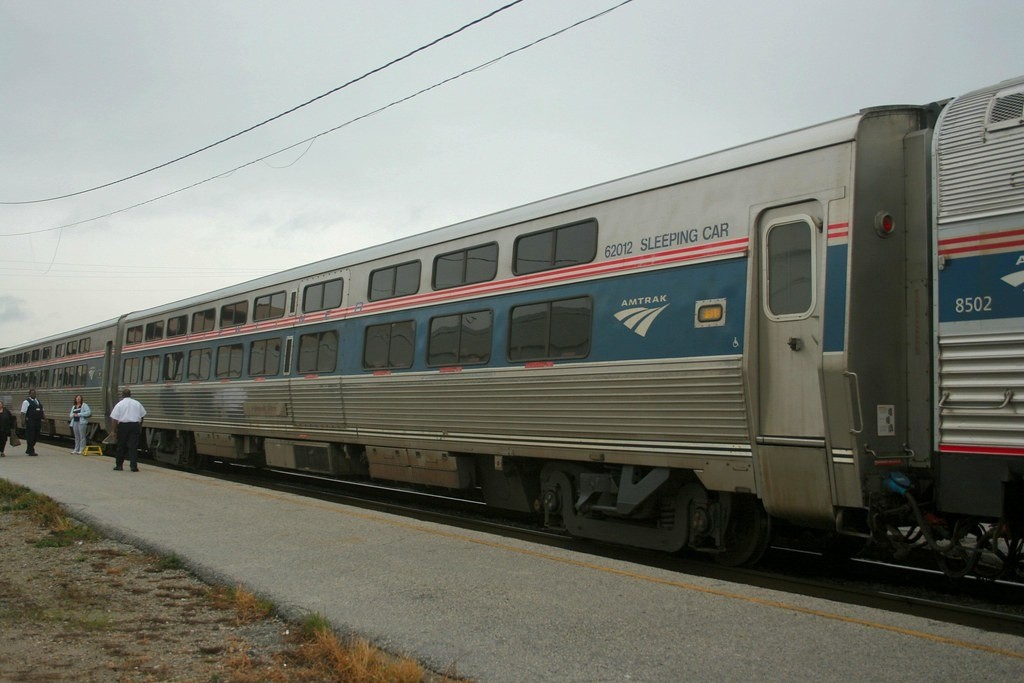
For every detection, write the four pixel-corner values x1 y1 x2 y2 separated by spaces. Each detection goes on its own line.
111 432 114 434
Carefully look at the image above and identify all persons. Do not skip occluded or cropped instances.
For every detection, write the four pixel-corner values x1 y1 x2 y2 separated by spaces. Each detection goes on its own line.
69 394 91 454
110 389 147 471
0 401 15 456
21 390 46 456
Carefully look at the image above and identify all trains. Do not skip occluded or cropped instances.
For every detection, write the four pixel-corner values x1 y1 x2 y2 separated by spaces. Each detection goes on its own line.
0 74 1024 635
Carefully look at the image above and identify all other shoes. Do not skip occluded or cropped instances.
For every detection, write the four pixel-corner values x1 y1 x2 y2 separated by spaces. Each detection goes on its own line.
25 450 38 456
1 453 5 457
113 466 123 471
131 467 139 472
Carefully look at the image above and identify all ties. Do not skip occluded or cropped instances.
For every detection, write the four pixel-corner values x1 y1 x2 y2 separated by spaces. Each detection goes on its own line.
34 399 37 404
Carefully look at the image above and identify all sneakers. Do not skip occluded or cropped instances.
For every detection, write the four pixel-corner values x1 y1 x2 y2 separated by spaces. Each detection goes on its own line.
70 452 82 455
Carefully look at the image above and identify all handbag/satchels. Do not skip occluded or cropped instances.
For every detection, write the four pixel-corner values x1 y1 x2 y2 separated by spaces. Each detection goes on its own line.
9 428 21 447
83 403 92 418
